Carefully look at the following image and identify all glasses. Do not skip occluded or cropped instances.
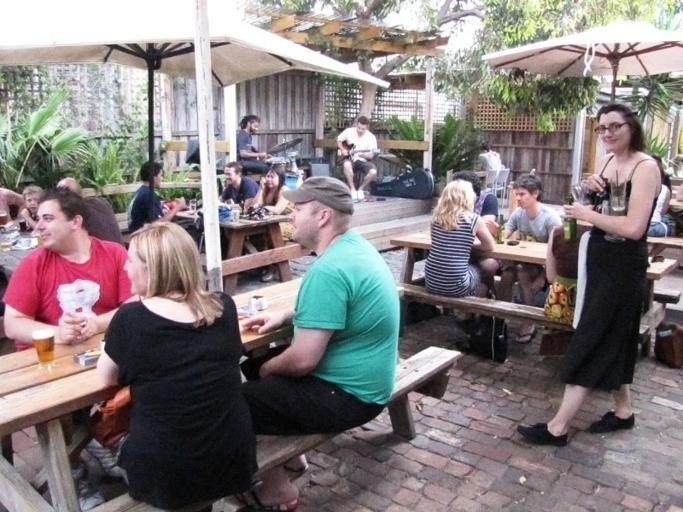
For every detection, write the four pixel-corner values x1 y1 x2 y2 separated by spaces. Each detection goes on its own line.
595 122 628 134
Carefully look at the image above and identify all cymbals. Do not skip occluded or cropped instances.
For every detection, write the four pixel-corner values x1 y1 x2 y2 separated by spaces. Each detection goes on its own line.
267 137 303 155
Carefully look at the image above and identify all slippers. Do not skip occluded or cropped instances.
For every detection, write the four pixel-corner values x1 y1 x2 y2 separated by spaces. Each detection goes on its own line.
235 481 299 512
284 460 309 474
513 327 537 343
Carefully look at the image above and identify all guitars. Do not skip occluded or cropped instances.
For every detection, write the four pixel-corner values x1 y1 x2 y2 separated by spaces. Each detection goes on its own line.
335 139 382 166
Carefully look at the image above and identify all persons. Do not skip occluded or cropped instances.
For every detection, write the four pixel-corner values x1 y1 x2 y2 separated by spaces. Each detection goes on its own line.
97 222 259 512
125 161 185 226
477 140 500 183
243 177 399 511
219 164 257 214
501 175 561 343
59 177 126 248
14 184 44 230
517 104 660 449
3 188 132 511
238 114 273 173
654 153 674 200
424 180 499 323
253 167 296 281
675 178 683 203
451 171 501 299
648 185 670 264
336 114 378 204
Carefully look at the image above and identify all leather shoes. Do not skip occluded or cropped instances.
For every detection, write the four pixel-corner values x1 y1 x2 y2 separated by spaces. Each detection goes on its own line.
588 412 634 433
516 423 568 447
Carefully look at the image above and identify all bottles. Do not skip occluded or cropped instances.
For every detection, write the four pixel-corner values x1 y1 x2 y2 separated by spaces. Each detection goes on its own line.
564 195 576 242
595 177 610 214
498 214 505 244
189 192 196 215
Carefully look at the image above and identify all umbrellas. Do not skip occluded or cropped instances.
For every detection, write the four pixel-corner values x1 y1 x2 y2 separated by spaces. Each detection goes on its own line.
480 19 683 102
0 2 390 159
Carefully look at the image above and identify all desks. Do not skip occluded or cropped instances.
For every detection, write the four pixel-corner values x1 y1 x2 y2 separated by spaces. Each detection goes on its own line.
390 233 677 356
0 228 41 276
0 276 309 512
668 198 683 212
175 210 292 293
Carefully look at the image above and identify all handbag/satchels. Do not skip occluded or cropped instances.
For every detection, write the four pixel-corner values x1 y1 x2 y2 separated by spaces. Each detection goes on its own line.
472 315 509 363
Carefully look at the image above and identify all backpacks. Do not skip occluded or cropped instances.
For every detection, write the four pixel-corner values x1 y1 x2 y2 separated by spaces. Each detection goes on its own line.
464 313 506 364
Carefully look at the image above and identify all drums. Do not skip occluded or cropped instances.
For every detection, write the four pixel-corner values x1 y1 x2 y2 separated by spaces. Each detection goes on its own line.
263 156 288 175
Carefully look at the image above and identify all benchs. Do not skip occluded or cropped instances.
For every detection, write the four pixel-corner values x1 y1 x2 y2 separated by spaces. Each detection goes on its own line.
122 235 210 265
86 346 463 512
576 219 683 250
653 288 681 304
399 282 649 343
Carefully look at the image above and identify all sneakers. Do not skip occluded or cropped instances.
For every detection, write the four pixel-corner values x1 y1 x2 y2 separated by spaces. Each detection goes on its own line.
358 190 364 201
261 266 273 281
79 479 105 511
351 190 357 202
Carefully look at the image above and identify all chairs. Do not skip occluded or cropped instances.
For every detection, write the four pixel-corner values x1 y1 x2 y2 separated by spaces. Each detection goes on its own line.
485 170 497 196
495 168 510 207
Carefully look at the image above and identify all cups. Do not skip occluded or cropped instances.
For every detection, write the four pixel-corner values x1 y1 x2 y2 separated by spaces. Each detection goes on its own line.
0 210 8 228
19 221 26 233
571 184 584 206
190 198 197 215
1 235 12 252
611 177 625 213
31 329 55 365
597 200 626 243
233 209 240 223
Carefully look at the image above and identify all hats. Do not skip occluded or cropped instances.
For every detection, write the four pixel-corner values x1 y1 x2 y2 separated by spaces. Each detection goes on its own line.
282 176 354 215
